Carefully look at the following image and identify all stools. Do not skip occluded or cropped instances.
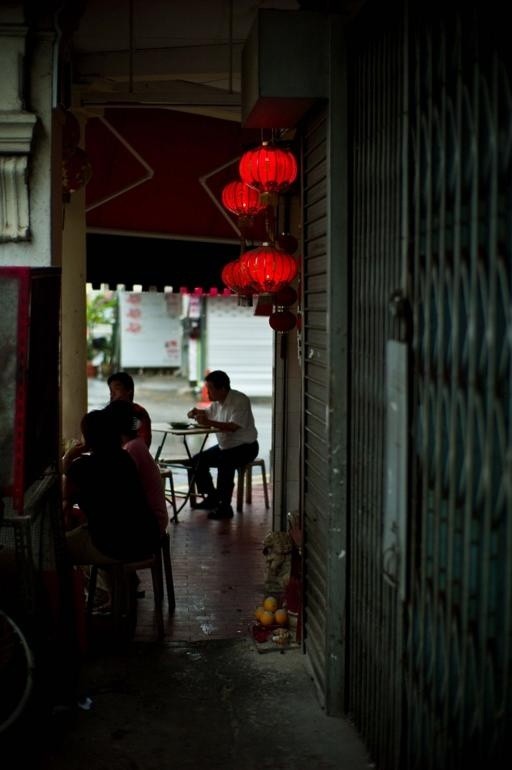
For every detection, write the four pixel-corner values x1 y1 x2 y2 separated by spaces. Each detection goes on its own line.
160 469 178 524
237 458 269 512
159 533 177 610
86 557 165 641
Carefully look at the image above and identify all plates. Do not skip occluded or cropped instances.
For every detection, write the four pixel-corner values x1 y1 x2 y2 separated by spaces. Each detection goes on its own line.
192 424 212 427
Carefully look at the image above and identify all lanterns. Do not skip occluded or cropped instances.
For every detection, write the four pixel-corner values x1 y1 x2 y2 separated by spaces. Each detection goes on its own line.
221 241 298 334
220 140 297 229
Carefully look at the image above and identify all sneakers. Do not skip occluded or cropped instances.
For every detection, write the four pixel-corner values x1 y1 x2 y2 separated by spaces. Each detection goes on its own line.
206 503 234 518
196 495 218 509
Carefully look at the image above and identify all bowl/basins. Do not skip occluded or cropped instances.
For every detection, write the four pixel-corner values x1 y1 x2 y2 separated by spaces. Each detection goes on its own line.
169 422 191 429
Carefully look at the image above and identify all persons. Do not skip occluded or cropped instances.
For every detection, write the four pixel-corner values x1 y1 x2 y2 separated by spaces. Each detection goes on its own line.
61 371 170 614
187 370 259 521
